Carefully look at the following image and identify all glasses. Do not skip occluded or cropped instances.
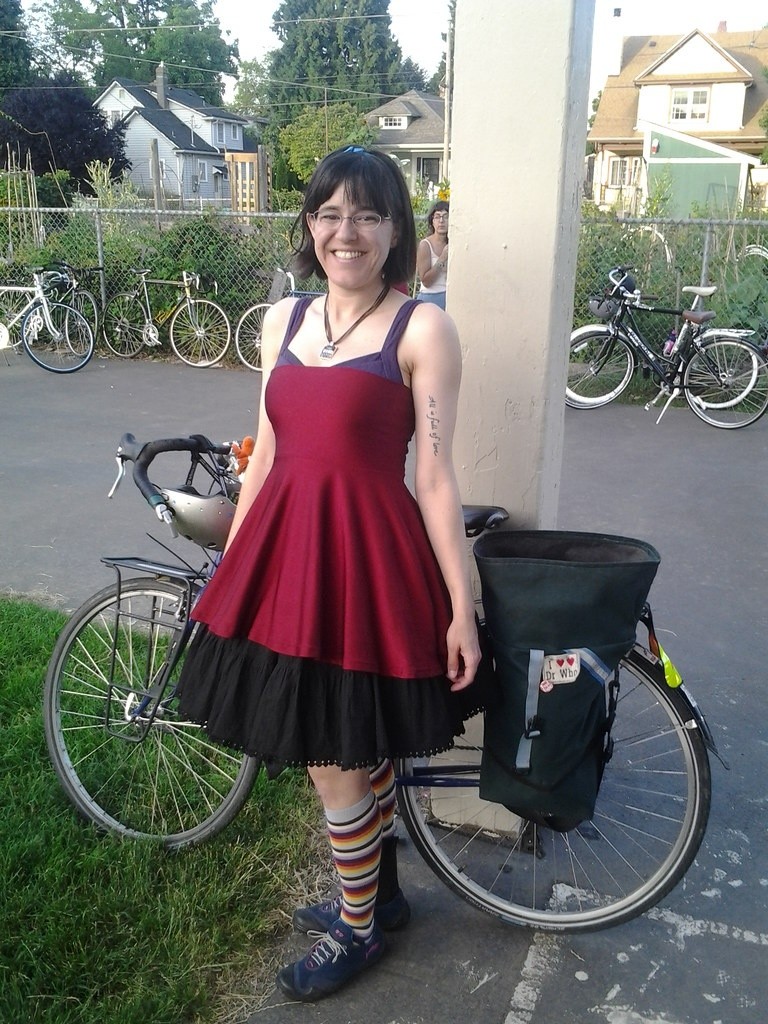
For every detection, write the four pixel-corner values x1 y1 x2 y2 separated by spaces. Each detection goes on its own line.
431 214 449 221
310 210 392 231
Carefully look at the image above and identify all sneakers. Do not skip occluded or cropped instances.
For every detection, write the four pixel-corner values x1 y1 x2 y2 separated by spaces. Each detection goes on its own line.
274 920 388 1003
292 888 412 935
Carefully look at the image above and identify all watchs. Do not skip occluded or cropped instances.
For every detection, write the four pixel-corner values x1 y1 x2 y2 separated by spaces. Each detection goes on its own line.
435 261 446 270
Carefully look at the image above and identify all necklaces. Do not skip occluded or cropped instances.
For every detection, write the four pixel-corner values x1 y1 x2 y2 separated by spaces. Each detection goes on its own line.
320 278 389 356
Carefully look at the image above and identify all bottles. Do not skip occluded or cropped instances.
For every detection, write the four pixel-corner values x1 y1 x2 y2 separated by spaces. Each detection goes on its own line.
663 331 676 353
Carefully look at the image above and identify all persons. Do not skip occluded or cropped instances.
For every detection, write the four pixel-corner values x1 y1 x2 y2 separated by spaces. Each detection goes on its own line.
414 198 450 312
178 142 482 1000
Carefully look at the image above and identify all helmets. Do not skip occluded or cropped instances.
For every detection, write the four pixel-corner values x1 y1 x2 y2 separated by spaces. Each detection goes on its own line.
588 295 621 319
161 484 241 551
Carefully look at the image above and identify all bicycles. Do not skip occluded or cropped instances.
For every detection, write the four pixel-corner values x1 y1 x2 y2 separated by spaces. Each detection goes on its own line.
234 267 327 373
0 267 94 374
42 432 733 932
564 263 768 429
102 267 231 369
1 260 105 358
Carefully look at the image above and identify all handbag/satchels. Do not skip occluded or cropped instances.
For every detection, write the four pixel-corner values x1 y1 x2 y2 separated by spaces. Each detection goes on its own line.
473 530 661 832
